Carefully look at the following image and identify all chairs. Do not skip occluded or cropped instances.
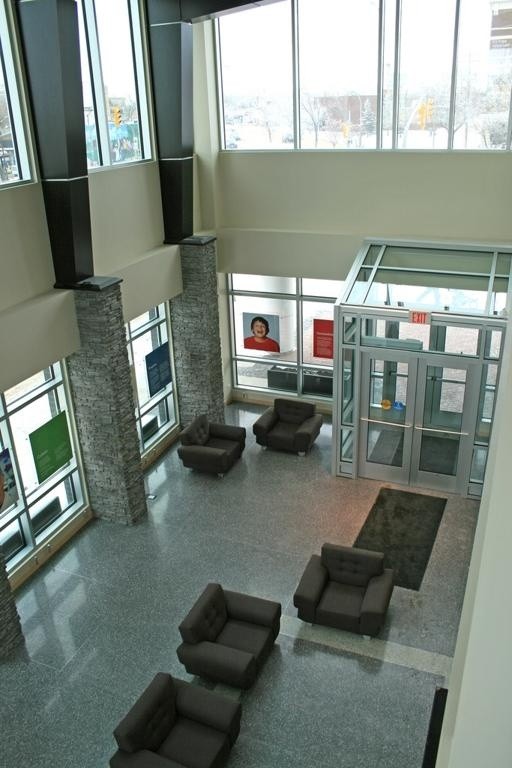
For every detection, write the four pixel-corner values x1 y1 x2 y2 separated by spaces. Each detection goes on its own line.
177 398 324 479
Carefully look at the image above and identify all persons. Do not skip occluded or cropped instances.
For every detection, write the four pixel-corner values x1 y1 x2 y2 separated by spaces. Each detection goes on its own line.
244 317 279 352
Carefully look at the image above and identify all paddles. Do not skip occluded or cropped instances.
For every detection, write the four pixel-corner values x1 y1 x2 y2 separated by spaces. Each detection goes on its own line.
349 485 448 591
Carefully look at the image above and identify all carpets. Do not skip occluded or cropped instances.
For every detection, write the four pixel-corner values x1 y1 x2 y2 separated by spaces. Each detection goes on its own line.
349 485 448 591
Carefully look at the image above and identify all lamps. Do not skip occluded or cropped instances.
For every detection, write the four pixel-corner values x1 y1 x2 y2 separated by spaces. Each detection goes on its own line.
177 398 324 479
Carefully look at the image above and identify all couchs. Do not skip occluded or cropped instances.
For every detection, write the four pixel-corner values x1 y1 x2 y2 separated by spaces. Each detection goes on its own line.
175 582 282 689
293 544 395 636
111 672 242 768
